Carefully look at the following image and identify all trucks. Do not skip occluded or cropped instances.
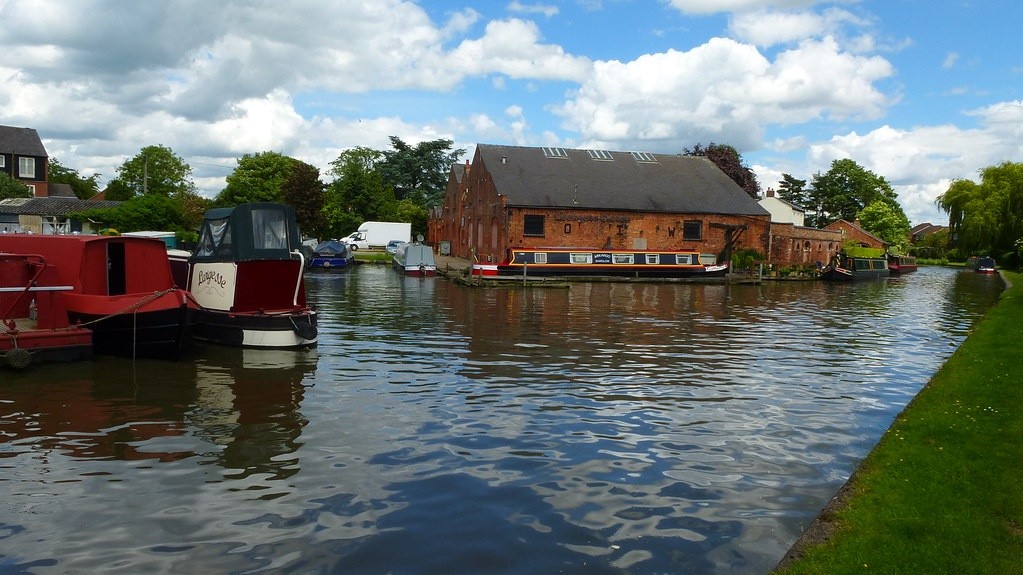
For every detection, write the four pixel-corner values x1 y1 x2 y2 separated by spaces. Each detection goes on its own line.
345 222 411 251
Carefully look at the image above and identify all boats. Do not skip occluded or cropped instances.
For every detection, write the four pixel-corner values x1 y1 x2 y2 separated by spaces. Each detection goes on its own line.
0 234 187 349
166 202 317 348
308 240 358 270
978 266 995 275
392 242 437 277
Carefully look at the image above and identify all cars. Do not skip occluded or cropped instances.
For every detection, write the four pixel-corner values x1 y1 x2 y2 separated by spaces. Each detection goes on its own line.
386 240 405 254
